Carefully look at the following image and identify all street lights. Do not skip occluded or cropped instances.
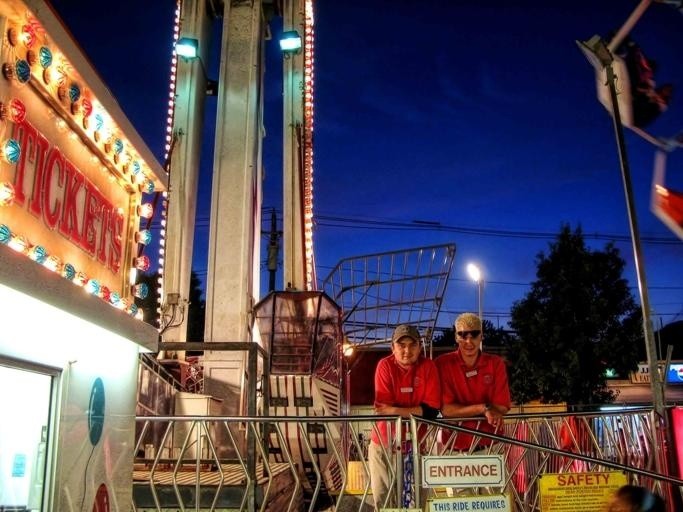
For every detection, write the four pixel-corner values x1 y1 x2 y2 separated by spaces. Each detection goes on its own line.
467 262 483 352
343 327 355 461
577 34 672 480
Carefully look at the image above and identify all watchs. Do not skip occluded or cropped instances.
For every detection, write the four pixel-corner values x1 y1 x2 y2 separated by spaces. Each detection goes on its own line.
483 403 490 413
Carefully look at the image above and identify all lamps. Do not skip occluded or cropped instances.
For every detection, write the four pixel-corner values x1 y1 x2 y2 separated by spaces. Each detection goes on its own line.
277 30 301 58
0 58 31 206
6 24 151 194
130 203 153 298
0 222 145 321
174 37 198 59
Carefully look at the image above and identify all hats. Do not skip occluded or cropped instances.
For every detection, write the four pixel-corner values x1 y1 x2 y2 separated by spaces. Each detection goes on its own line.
392 325 420 341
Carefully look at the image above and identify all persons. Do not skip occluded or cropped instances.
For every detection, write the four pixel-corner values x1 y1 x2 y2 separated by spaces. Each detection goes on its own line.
539 416 557 467
435 313 511 512
606 487 668 512
370 325 440 512
614 428 633 470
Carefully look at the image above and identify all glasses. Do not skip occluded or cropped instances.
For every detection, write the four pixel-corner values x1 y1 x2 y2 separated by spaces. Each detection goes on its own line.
455 330 480 338
